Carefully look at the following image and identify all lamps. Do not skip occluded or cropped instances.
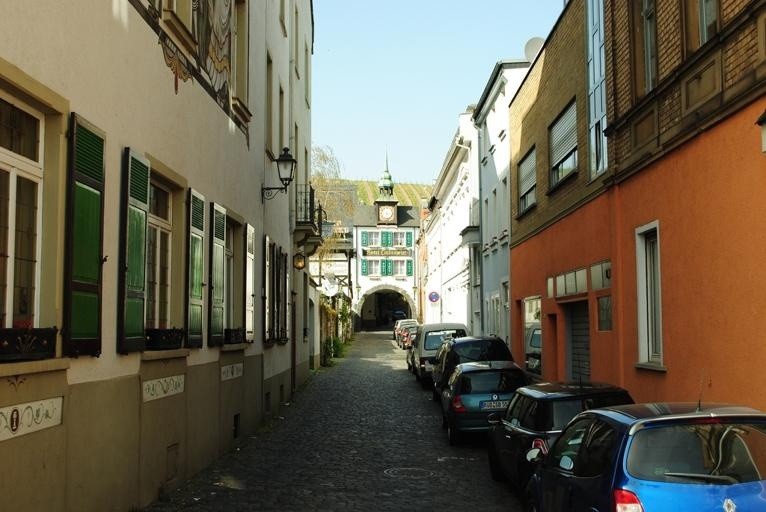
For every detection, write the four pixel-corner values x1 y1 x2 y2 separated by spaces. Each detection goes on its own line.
293 253 305 270
261 147 297 203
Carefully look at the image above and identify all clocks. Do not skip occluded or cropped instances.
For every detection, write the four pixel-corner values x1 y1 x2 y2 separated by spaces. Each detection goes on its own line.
379 206 394 221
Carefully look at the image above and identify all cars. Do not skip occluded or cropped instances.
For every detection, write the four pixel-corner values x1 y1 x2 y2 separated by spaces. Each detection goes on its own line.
486 378 635 503
521 389 766 512
389 311 542 446
